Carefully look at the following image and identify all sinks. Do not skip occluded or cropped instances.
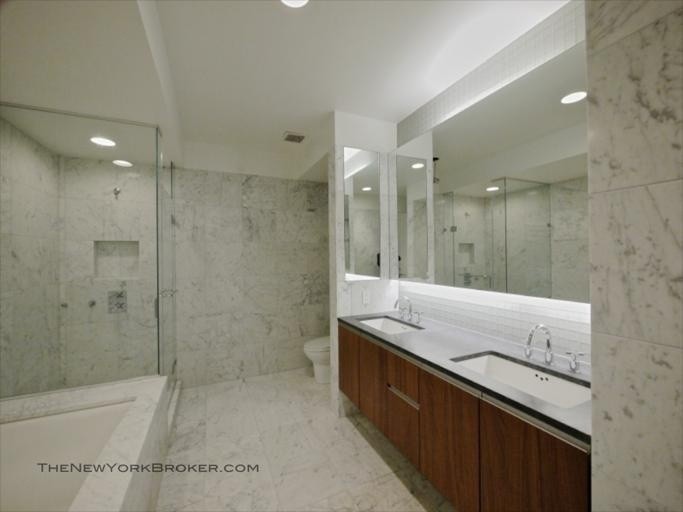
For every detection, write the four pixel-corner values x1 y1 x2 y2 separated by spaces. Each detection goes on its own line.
357 314 425 337
448 348 591 411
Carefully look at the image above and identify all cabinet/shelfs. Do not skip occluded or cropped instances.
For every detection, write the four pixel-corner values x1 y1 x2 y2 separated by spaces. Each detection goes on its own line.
339 316 591 512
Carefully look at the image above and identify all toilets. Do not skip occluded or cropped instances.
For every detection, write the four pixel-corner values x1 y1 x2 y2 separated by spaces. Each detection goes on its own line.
304 337 331 383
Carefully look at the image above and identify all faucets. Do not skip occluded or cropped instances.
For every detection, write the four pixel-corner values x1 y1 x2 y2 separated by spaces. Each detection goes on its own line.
524 323 553 369
392 294 415 321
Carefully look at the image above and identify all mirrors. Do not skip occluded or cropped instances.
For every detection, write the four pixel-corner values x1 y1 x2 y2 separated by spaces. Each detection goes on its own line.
341 37 590 304
